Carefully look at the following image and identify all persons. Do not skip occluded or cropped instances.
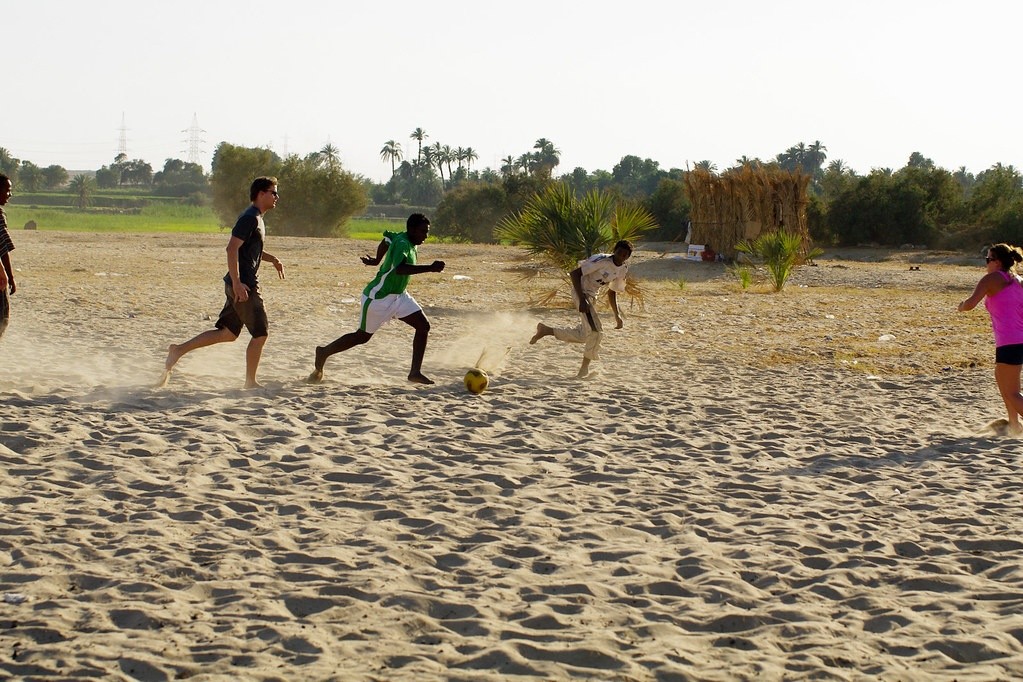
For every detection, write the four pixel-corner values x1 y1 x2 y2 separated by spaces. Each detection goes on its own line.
166 177 285 389
530 240 633 375
0 174 16 337
315 214 446 384
958 245 1023 429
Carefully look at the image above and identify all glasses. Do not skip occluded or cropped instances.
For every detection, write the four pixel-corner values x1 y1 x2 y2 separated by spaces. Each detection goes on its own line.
266 189 279 196
986 257 997 264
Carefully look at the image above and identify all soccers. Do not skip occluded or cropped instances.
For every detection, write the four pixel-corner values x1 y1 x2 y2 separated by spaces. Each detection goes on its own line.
464 368 489 394
